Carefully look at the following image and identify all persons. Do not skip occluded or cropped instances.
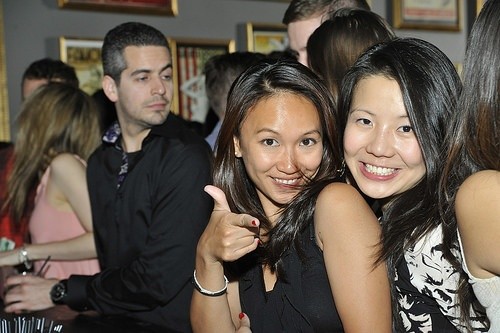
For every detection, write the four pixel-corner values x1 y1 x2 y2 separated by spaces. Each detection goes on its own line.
0 59 117 326
189 51 396 333
337 38 500 333
204 0 500 227
4 22 214 333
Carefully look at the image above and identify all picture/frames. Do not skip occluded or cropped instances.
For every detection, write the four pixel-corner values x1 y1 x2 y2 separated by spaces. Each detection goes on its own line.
58 0 180 18
59 34 109 95
239 21 293 59
169 35 239 121
390 0 464 32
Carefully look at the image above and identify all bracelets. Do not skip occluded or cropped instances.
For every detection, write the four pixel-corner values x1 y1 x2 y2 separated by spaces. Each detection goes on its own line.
192 268 228 297
21 244 32 269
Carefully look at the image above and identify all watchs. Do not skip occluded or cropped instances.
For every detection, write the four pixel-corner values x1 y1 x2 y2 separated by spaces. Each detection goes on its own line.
49 279 69 305
